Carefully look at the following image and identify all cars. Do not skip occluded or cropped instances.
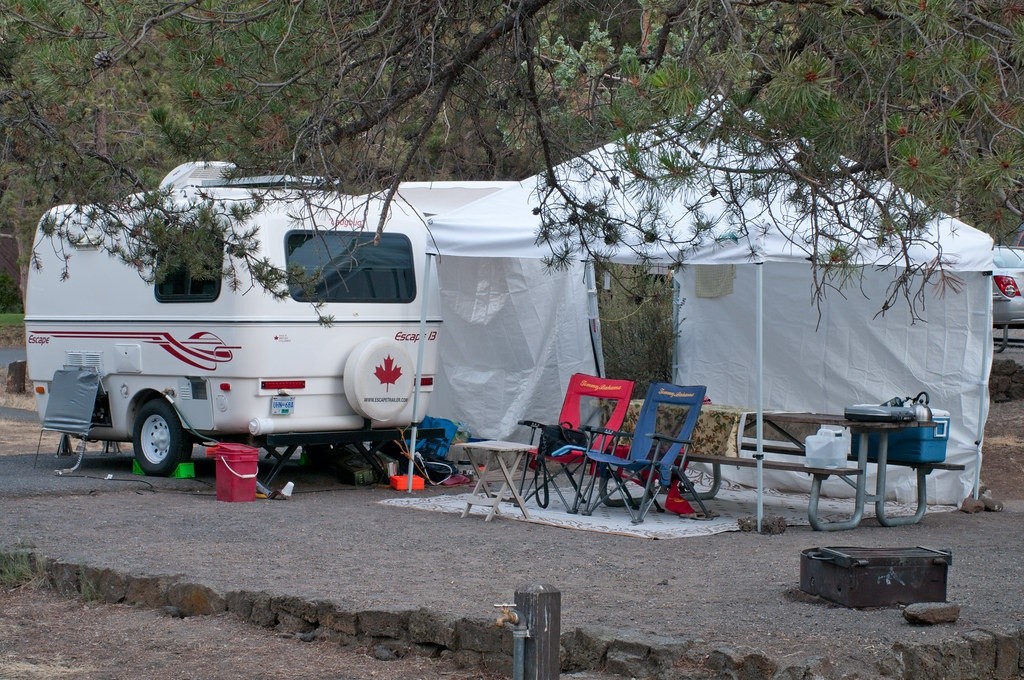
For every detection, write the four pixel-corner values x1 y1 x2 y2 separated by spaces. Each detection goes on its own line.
993 245 1024 327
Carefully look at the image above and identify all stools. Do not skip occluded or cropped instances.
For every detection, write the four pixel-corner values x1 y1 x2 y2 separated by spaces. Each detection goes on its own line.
455 441 538 522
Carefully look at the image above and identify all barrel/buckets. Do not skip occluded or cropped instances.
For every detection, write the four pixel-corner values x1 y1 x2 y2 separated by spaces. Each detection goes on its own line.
215 443 258 502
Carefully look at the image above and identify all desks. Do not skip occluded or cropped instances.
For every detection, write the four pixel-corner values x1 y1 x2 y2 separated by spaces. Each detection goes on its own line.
607 398 940 526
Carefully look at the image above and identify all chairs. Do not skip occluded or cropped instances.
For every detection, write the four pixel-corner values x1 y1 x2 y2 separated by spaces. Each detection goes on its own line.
580 378 715 525
513 373 635 514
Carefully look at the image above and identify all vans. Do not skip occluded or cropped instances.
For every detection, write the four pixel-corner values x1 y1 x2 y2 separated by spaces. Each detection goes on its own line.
25 159 446 480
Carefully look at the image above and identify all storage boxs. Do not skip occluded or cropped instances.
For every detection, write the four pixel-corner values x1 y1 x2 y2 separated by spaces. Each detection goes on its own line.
332 451 399 486
850 403 951 462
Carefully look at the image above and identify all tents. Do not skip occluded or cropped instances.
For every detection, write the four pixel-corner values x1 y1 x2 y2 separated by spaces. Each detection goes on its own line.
406 94 998 532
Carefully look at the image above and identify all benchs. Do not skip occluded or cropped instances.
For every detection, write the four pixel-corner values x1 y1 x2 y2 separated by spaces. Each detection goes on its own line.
597 445 863 529
740 441 964 529
